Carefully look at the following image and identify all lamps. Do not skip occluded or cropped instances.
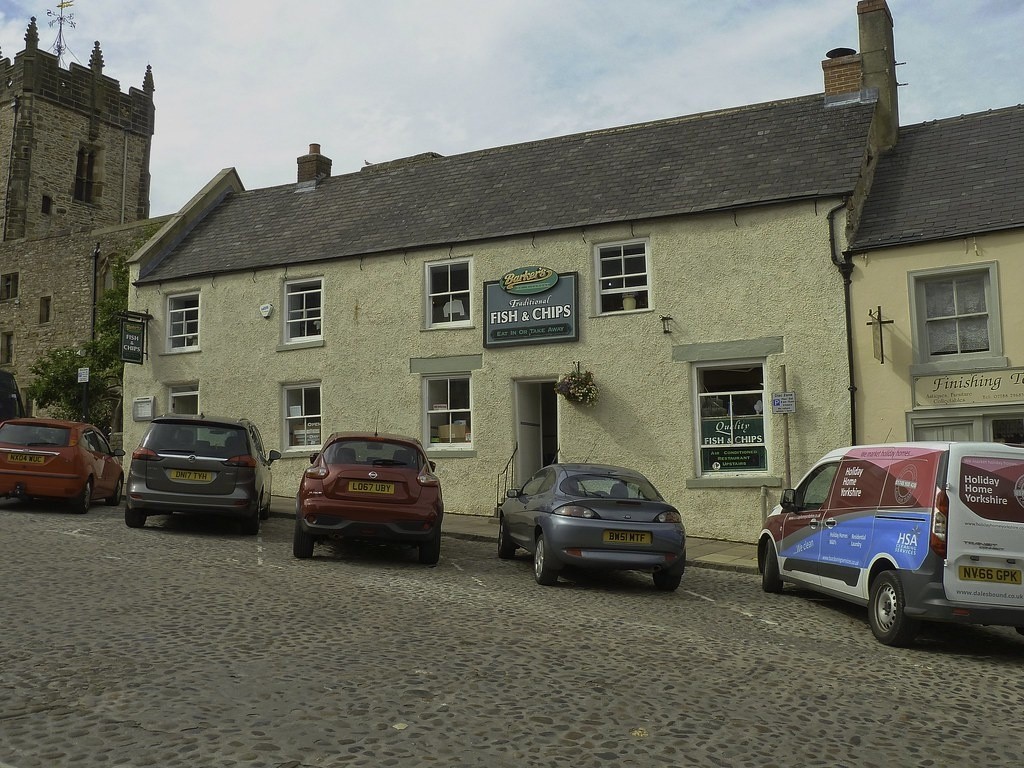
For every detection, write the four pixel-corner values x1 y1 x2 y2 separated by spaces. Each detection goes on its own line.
659 314 673 332
621 291 639 311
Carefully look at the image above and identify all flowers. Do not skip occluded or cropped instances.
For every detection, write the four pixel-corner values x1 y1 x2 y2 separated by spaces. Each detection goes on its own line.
553 369 599 408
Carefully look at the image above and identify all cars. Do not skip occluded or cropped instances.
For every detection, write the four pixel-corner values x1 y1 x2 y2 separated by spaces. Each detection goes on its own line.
500 464 687 591
294 429 445 565
1 417 123 516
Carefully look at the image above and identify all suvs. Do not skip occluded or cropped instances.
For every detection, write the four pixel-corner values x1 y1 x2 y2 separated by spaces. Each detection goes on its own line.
124 413 281 535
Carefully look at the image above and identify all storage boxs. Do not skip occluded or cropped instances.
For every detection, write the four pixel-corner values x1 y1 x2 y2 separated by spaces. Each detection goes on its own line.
438 424 471 442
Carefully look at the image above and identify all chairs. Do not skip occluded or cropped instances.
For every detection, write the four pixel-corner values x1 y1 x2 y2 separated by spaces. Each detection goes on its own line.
177 430 192 446
225 436 244 452
561 478 578 495
392 450 411 465
609 483 629 499
336 447 356 463
195 440 209 447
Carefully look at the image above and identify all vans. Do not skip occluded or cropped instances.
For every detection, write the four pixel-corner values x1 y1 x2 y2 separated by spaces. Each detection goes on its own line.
756 440 1024 648
